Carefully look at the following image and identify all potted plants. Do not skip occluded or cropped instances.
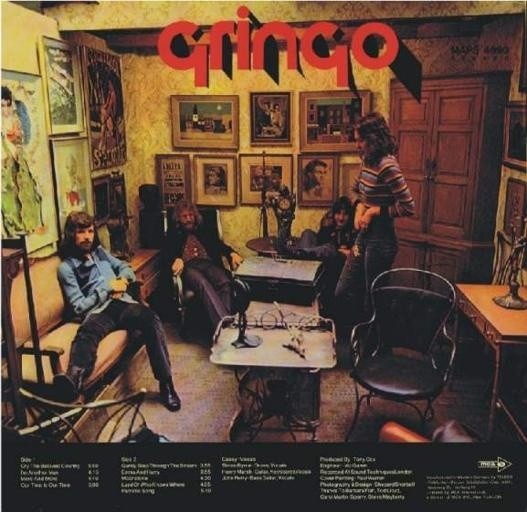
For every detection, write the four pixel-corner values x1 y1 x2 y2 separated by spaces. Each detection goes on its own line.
341 161 362 200
82 43 136 168
494 165 525 276
1 66 62 254
36 32 87 135
298 88 370 155
249 91 292 145
52 137 94 240
154 153 190 204
504 103 527 168
296 156 340 209
519 27 527 92
192 154 237 208
238 153 294 206
168 93 241 152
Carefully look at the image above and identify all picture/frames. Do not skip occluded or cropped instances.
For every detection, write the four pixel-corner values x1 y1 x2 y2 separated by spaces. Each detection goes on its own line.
450 279 527 439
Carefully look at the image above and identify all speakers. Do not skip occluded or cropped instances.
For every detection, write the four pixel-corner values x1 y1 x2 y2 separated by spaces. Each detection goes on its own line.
160 376 181 411
52 365 86 402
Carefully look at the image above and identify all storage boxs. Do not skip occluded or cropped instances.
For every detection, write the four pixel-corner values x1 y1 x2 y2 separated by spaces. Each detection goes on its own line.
4 239 155 435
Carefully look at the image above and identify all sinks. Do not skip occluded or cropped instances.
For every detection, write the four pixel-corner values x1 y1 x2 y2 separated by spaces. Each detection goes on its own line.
373 420 427 442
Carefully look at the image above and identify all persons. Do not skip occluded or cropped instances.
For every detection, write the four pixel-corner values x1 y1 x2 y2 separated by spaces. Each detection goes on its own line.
251 166 279 192
206 168 226 195
334 111 415 319
302 159 330 198
171 199 242 326
1 86 44 239
319 195 356 287
264 103 283 126
53 210 181 412
99 80 118 147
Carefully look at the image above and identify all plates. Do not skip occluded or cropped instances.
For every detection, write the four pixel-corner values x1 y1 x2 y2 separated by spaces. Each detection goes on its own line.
385 79 513 242
375 247 473 281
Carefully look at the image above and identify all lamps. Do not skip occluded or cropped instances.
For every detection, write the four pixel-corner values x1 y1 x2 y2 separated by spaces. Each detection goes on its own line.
344 266 460 440
